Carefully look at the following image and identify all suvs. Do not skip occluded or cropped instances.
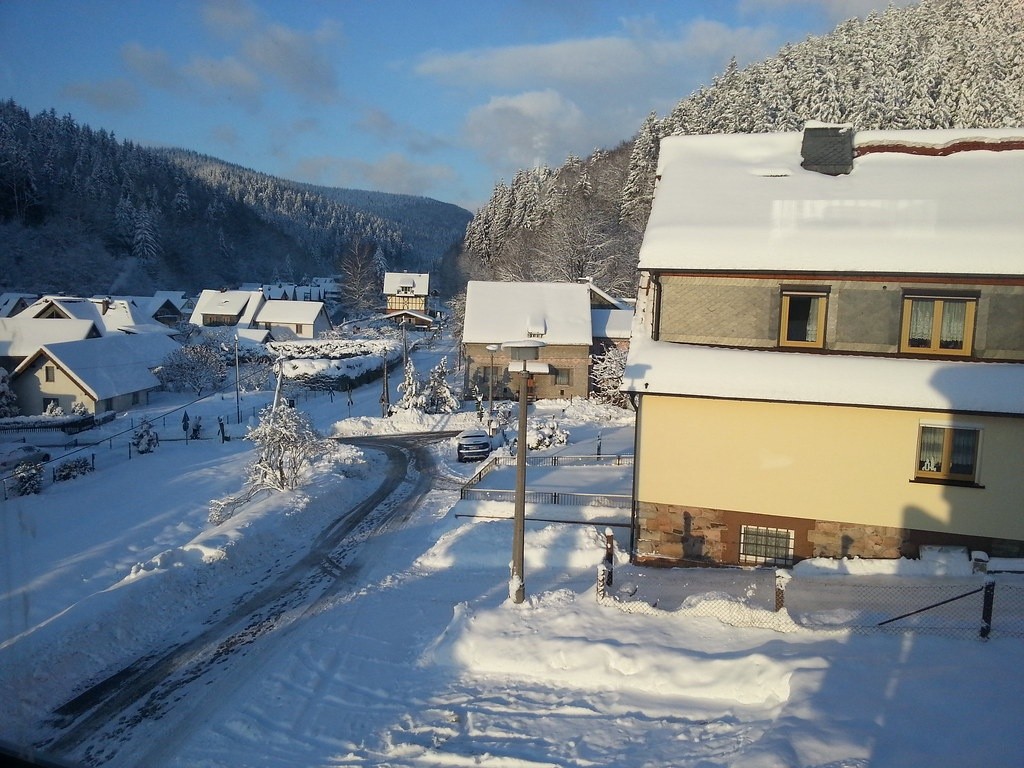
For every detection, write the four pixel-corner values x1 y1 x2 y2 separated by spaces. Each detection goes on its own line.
456 430 494 462
